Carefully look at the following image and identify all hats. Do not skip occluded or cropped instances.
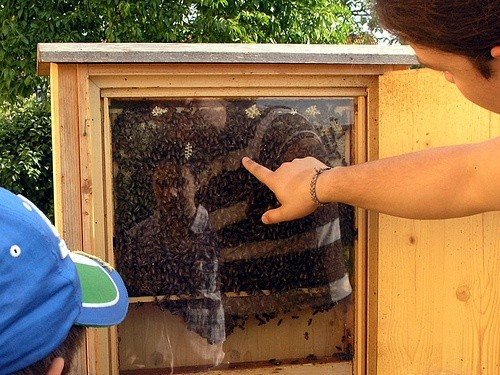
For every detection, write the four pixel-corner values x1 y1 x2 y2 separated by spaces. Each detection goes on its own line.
0 185 130 375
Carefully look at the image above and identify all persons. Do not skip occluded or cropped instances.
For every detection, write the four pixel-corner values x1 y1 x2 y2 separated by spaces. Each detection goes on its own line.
0 189 90 375
122 148 226 360
242 0 500 224
172 103 352 320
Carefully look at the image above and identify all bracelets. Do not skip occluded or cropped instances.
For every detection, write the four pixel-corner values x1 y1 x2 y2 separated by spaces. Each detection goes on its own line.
309 165 332 207
